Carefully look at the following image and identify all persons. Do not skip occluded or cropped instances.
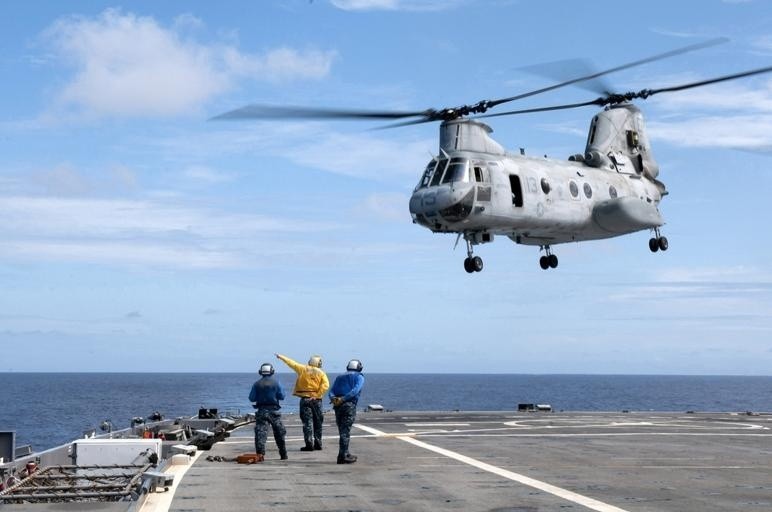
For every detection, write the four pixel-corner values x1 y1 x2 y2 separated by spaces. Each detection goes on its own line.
328 359 365 464
248 362 289 463
273 351 330 452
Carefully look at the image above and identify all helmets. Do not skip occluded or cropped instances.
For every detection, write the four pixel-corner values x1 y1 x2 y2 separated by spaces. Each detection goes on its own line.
347 358 363 373
258 362 275 376
308 353 324 367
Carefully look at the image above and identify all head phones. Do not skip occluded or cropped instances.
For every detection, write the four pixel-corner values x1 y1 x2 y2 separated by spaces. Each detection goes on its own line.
308 359 322 366
259 363 275 374
346 359 363 371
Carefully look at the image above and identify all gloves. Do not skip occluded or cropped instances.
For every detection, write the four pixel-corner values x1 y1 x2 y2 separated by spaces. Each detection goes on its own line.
329 396 343 407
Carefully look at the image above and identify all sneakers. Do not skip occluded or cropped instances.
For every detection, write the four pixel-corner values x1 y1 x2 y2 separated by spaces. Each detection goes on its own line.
300 445 323 451
280 453 289 460
337 453 357 464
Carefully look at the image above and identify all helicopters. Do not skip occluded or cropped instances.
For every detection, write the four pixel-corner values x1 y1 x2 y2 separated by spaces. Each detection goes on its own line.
211 35 771 277
